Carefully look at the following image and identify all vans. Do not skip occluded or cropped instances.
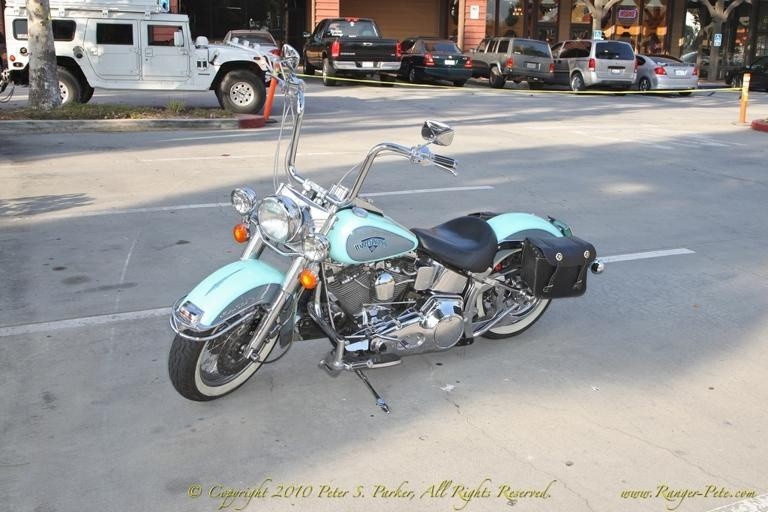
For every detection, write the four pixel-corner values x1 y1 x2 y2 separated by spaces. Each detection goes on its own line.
547 38 636 95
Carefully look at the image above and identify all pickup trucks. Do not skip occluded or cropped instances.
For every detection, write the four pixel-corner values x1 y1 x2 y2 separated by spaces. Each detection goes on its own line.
300 15 402 88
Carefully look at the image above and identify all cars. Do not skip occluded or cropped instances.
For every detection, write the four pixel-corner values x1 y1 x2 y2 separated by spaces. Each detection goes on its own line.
724 56 768 93
631 54 700 96
222 29 282 74
399 36 474 87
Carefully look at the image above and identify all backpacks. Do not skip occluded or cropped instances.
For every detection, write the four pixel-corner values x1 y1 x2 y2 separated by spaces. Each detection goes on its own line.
519 234 597 299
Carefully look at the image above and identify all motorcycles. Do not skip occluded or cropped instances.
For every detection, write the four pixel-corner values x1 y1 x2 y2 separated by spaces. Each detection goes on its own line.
168 44 605 417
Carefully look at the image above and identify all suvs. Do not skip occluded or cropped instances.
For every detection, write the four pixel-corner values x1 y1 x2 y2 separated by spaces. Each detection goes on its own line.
470 36 555 89
0 0 275 116
